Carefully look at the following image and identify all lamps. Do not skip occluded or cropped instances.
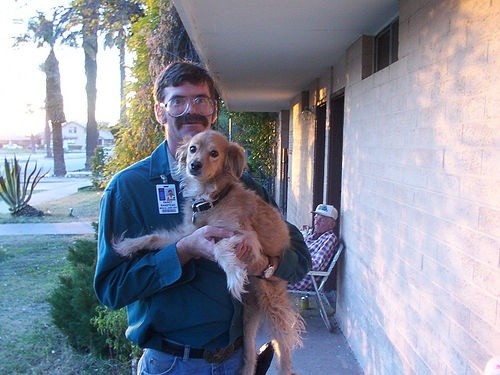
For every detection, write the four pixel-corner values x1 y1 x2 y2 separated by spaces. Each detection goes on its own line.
299 107 316 120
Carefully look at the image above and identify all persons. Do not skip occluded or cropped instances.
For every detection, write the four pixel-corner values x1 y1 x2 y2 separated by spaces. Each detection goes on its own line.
93 62 311 375
286 204 338 291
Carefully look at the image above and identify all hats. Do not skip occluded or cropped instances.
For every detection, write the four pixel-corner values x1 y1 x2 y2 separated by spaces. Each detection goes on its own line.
311 204 339 221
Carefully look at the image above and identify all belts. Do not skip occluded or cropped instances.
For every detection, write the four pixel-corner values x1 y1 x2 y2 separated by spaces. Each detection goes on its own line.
144 337 244 362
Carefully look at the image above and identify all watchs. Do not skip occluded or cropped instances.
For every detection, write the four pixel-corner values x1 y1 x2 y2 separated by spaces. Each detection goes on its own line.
256 255 275 279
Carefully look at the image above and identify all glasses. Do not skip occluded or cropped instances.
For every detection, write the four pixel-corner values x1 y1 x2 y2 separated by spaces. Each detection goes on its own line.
160 96 215 117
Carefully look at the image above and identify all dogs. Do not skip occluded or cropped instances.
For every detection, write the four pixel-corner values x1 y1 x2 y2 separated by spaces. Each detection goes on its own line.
111 130 308 375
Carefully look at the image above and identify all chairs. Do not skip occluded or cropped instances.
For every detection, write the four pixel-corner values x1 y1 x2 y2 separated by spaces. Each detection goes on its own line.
285 241 344 333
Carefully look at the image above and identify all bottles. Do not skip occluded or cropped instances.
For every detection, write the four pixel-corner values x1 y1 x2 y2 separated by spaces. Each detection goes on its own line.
300 297 309 309
